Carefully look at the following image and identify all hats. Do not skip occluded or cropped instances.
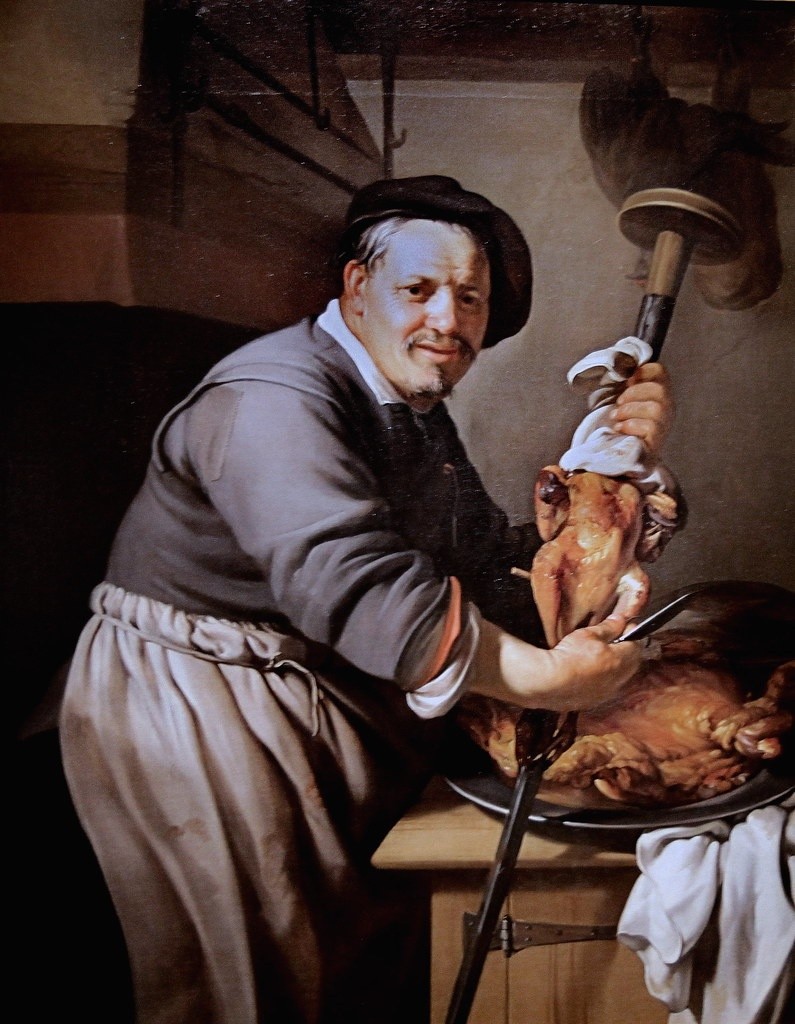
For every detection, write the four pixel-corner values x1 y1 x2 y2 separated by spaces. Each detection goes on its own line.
336 175 533 349
